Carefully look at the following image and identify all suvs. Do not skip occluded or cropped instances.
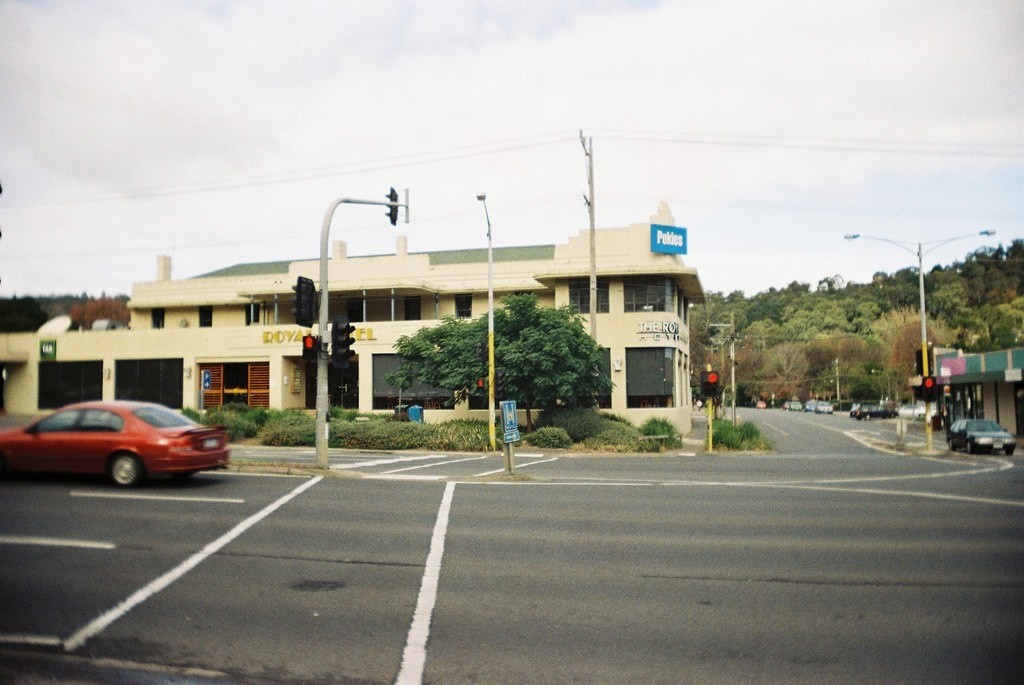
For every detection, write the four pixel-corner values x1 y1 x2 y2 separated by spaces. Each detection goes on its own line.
850 401 899 421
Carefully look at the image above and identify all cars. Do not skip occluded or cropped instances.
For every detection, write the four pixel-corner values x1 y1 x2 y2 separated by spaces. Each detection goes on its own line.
783 399 834 415
756 400 766 410
946 418 1017 456
0 398 232 491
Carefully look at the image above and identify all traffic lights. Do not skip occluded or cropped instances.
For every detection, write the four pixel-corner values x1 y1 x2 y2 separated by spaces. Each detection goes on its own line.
386 187 399 226
331 315 356 370
302 336 315 352
700 371 720 397
290 276 316 328
922 377 938 401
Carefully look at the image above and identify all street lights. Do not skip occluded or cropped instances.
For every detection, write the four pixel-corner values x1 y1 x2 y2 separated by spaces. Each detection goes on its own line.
476 190 496 452
843 228 997 458
871 369 891 402
